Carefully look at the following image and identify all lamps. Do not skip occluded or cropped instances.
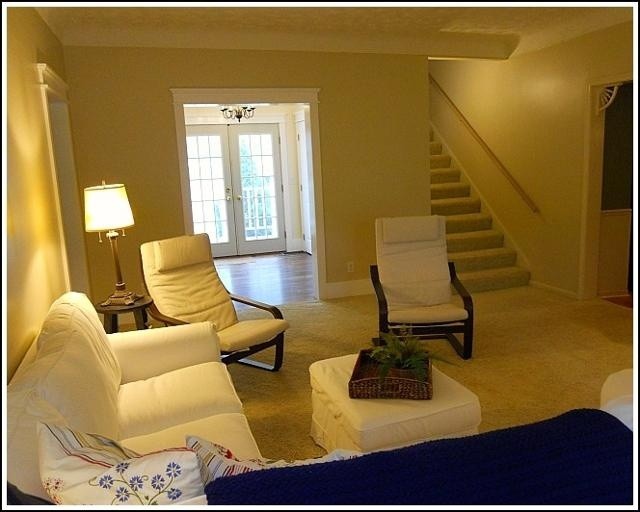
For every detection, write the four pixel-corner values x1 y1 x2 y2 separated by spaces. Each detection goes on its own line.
216 102 256 123
81 180 144 307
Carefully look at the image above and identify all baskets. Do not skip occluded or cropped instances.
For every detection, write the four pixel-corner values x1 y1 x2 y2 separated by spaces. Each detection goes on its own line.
348 348 433 400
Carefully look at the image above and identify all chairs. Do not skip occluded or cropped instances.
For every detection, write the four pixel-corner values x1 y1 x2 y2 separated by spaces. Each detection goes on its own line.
368 214 474 359
138 233 291 373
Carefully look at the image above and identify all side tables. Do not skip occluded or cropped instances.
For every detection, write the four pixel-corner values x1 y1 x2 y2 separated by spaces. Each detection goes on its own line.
95 295 154 334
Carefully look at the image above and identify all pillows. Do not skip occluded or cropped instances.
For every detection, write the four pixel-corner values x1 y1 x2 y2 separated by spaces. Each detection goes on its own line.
184 421 359 486
33 421 208 505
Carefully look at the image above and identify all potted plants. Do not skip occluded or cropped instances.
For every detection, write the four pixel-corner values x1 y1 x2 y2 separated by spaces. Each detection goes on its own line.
364 320 463 395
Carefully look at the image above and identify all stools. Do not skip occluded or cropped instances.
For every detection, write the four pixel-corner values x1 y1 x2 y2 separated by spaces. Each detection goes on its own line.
308 355 483 458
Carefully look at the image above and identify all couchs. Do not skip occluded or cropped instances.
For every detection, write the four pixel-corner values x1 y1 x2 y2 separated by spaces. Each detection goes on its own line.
5 289 266 505
